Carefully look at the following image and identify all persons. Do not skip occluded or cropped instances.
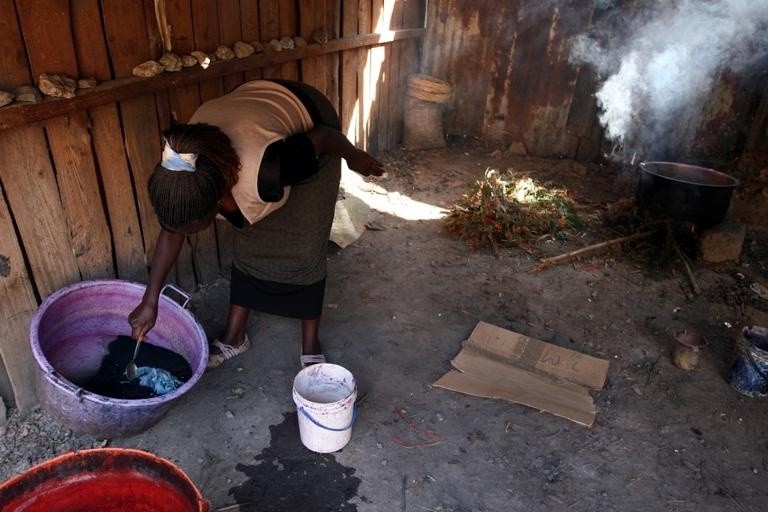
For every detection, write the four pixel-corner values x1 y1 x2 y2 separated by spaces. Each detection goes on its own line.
125 78 387 373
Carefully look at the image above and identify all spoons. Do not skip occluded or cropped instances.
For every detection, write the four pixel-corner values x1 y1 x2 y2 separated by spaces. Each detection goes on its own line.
126 338 142 379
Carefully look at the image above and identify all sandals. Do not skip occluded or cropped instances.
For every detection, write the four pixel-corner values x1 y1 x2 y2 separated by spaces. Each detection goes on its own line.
204 332 251 369
299 334 327 369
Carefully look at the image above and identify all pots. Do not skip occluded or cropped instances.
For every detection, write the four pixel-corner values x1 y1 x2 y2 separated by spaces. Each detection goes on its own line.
636 161 740 229
29 278 211 437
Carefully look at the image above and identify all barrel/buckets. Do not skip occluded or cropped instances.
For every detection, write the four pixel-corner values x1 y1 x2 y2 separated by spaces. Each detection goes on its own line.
291 363 359 455
0 447 213 512
725 325 767 401
